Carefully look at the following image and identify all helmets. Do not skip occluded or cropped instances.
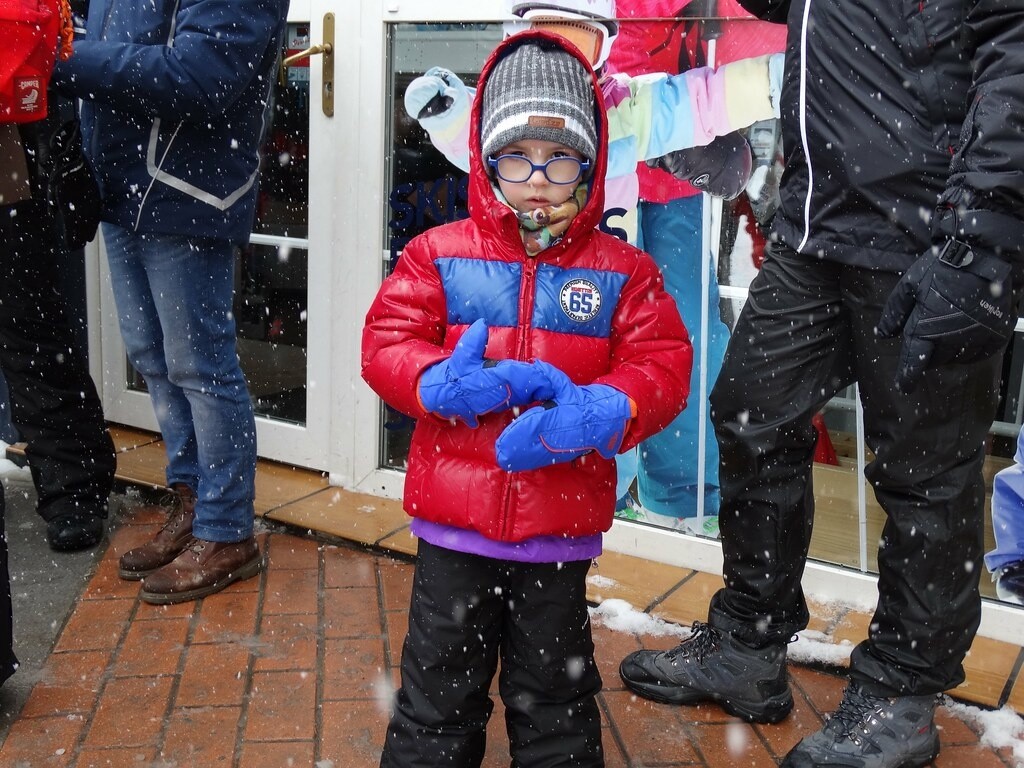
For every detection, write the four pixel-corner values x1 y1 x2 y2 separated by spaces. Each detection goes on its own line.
511 0 619 70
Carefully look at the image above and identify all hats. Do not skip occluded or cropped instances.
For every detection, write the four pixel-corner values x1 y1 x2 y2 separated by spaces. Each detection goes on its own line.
480 42 598 180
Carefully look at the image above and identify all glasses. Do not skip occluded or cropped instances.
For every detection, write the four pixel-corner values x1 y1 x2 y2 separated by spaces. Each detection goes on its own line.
488 153 590 185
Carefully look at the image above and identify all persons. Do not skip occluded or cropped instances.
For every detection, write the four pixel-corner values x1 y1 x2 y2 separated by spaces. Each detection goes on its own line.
619 0 1024 768
0 0 289 702
364 31 694 768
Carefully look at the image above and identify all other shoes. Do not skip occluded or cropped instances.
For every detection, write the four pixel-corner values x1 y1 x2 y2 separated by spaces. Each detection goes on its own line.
47 512 104 553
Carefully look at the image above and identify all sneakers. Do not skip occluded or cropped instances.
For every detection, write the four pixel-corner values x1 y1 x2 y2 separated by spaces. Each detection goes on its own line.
779 681 940 768
619 620 795 725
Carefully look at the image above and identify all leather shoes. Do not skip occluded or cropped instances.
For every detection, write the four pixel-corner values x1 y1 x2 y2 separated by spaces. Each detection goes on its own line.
118 483 198 581
139 534 264 605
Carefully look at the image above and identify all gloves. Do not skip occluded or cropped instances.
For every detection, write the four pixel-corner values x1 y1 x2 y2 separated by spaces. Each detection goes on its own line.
416 317 555 430
495 358 638 472
34 118 103 250
404 76 457 120
878 237 1020 395
769 53 784 93
646 130 752 201
